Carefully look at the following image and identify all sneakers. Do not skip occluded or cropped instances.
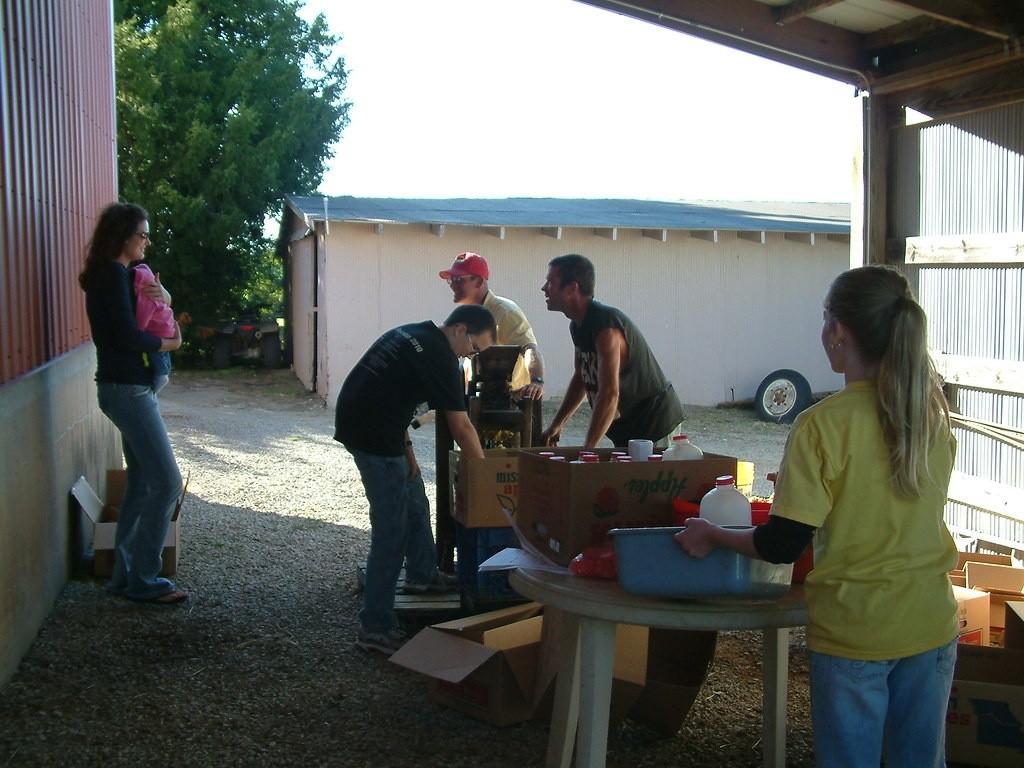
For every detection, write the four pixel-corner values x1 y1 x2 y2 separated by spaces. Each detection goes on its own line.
357 624 410 655
403 565 458 592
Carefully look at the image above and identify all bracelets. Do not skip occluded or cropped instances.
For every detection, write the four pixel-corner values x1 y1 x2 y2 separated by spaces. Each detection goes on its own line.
403 440 413 447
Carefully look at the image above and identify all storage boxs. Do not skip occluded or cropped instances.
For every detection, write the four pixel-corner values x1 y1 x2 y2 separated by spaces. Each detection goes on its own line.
70 469 191 577
946 552 1024 768
387 448 737 739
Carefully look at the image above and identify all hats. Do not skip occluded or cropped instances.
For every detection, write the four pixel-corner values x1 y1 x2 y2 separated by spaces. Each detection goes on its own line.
439 252 490 280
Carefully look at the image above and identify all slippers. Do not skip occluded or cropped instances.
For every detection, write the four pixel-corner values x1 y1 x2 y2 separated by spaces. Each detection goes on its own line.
139 591 187 604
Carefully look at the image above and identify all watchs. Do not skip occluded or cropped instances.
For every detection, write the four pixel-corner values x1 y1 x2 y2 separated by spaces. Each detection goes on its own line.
530 376 545 384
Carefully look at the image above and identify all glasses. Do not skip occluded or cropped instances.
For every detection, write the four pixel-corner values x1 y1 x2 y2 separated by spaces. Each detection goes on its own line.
447 273 478 284
134 231 149 239
464 329 480 357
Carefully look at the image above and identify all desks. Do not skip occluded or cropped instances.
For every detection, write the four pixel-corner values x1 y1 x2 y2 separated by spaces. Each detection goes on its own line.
508 565 809 768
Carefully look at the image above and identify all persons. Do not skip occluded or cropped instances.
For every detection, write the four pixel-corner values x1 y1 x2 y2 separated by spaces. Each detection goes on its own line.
674 266 958 768
439 253 546 399
332 304 499 655
536 253 687 450
80 200 188 605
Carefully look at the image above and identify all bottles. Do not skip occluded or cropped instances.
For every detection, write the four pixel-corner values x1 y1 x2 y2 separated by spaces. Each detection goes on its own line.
700 475 752 528
539 435 704 463
